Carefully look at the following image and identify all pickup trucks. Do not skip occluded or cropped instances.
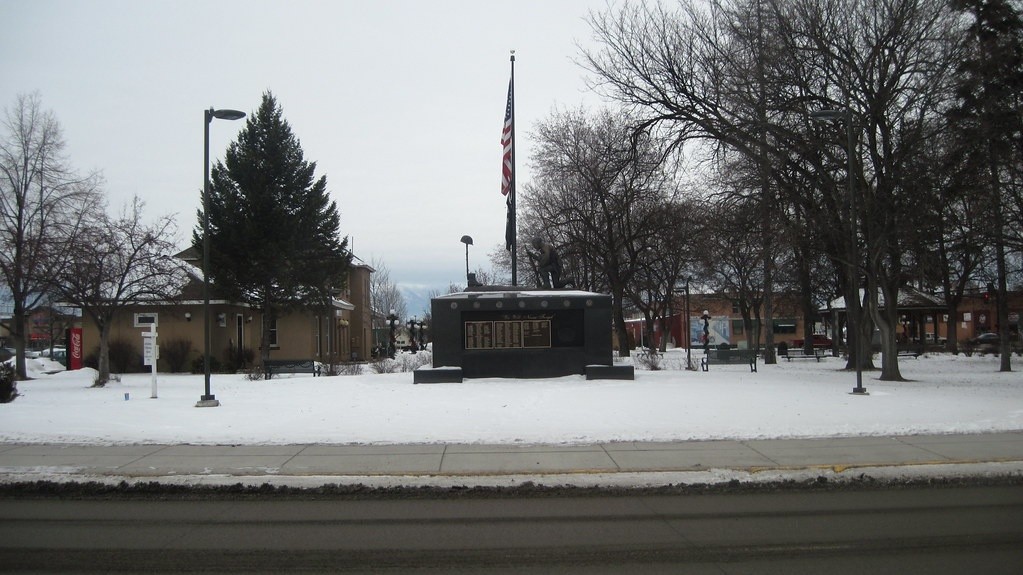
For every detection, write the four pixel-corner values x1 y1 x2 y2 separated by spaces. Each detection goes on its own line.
909 332 947 344
791 334 832 348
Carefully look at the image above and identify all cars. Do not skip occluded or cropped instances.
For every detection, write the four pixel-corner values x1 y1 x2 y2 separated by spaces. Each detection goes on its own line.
977 332 1000 341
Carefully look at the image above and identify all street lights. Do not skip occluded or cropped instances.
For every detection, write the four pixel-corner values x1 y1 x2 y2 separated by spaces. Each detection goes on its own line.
386 308 399 360
811 108 868 395
700 310 711 354
195 106 247 406
406 315 427 354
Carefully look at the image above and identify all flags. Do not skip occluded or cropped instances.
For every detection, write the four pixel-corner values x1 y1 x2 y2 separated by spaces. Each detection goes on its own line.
506 188 513 252
500 78 512 197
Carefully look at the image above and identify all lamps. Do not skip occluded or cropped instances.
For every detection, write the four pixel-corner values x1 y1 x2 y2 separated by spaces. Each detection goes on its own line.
246 316 253 324
337 319 349 329
185 312 192 320
215 312 225 322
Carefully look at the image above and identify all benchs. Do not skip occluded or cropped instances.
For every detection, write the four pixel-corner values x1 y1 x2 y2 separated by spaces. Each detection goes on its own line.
700 350 758 373
263 359 321 380
896 345 923 359
782 348 827 362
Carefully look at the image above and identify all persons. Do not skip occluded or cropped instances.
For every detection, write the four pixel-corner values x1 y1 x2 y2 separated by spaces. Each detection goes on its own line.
530 238 575 288
